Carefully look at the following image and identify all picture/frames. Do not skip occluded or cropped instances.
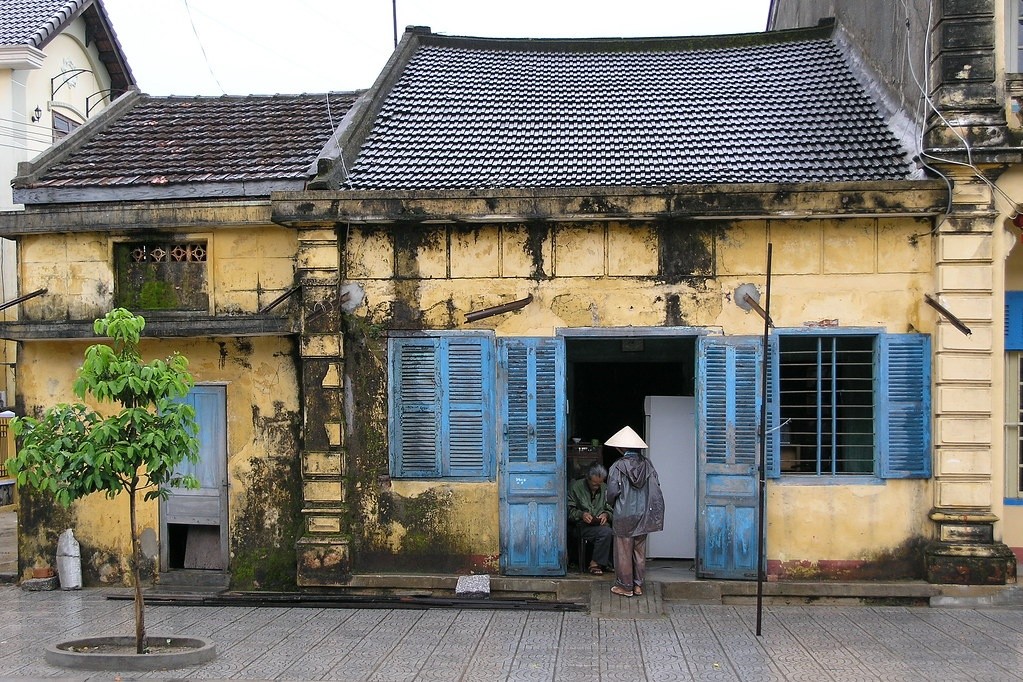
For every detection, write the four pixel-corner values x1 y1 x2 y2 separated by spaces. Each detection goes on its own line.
621 339 646 352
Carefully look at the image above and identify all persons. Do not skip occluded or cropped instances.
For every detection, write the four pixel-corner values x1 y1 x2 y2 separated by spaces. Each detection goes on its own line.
603 426 664 596
567 463 614 575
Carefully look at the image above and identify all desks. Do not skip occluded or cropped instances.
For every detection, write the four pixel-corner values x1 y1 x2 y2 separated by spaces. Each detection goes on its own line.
568 445 603 480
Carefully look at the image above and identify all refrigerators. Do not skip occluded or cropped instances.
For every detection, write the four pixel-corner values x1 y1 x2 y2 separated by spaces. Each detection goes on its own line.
640 395 699 559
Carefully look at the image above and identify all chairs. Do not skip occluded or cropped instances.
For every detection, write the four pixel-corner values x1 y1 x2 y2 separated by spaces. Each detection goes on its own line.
568 521 591 573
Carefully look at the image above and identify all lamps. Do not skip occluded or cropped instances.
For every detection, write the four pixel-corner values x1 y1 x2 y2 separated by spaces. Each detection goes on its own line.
32 104 42 123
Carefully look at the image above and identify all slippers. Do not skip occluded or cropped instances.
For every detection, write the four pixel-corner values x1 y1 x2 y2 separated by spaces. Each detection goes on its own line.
600 565 616 572
611 585 634 597
634 584 643 595
588 563 603 576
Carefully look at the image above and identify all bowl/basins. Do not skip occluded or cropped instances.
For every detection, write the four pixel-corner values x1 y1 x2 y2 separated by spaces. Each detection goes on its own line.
572 437 581 443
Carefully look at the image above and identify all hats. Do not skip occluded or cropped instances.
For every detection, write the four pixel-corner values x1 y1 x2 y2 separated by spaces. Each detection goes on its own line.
604 426 651 448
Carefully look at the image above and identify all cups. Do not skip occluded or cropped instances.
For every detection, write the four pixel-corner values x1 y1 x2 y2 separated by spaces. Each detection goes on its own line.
592 439 598 446
